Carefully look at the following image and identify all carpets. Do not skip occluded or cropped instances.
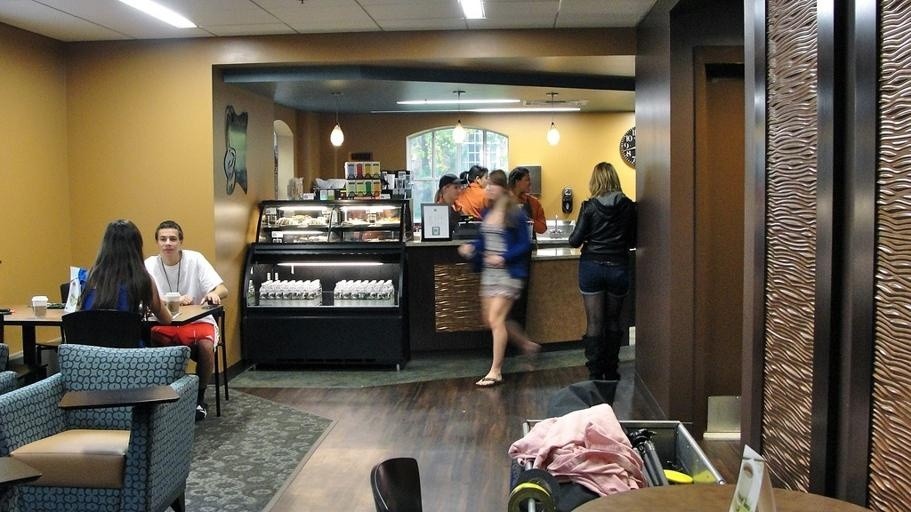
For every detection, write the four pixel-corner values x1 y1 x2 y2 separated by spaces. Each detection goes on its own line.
223 345 636 389
162 385 339 512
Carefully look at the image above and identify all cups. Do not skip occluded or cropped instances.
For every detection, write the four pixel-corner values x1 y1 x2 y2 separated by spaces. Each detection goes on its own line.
526 221 534 238
267 215 277 226
31 295 48 318
368 214 376 224
319 189 335 202
381 170 413 200
165 292 181 316
413 232 421 243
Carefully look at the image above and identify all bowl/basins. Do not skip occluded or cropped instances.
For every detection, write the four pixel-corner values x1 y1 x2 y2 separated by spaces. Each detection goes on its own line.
303 193 316 200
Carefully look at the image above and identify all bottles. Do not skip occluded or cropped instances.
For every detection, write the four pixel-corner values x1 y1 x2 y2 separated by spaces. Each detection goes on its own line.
294 178 303 200
248 280 256 306
259 272 322 300
333 279 395 299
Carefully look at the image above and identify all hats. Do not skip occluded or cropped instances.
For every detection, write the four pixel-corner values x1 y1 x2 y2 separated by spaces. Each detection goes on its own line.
439 174 464 188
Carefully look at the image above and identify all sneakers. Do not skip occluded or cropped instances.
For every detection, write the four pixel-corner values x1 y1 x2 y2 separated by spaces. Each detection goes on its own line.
195 404 207 421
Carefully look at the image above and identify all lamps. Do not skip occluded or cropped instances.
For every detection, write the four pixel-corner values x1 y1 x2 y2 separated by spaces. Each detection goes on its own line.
452 91 465 144
548 92 559 145
329 92 344 147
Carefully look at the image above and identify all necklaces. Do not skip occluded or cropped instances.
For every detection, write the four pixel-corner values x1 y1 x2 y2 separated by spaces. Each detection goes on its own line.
160 256 180 292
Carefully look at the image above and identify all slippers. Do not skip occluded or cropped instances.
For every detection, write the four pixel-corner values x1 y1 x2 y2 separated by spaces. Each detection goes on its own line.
474 377 505 387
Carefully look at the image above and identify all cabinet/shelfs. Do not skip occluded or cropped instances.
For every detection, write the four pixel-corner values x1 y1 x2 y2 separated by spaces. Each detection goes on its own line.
240 198 413 369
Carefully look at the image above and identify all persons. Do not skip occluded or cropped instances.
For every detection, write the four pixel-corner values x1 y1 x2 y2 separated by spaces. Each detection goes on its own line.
79 218 172 348
434 165 548 241
142 220 229 418
458 169 542 386
569 163 636 381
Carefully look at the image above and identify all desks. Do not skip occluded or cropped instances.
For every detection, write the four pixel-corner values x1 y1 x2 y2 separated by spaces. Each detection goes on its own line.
568 483 873 512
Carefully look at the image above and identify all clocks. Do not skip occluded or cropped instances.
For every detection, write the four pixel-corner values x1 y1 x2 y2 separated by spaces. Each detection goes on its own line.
620 127 635 169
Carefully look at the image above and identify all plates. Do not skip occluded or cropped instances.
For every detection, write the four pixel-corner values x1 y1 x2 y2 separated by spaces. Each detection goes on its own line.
278 224 329 228
341 221 401 228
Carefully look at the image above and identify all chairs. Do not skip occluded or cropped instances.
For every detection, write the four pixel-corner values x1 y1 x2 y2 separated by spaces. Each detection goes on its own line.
190 301 228 416
370 458 423 512
0 343 19 395
62 309 143 348
0 345 228 512
35 282 70 364
